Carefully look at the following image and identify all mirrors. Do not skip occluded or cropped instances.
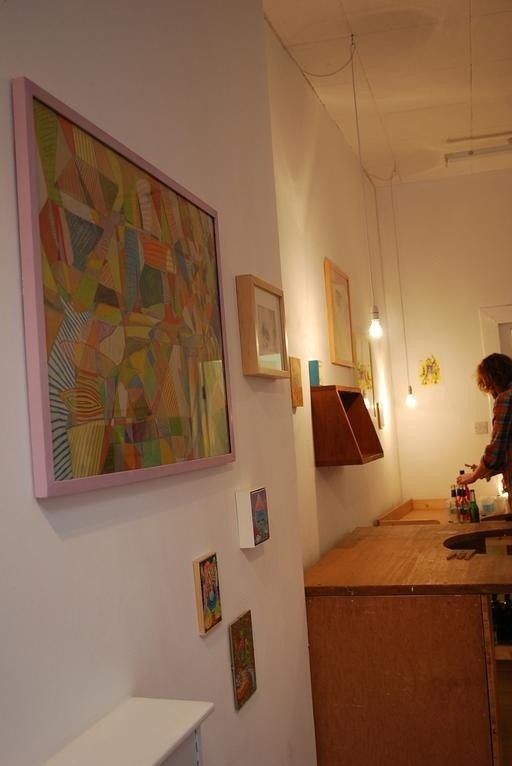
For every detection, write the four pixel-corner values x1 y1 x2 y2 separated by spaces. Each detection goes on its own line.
349 331 380 434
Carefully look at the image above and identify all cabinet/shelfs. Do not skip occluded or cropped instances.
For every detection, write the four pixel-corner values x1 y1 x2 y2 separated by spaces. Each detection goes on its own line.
36 694 219 765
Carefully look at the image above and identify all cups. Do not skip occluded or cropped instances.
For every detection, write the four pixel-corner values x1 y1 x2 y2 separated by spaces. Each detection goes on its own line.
479 494 507 514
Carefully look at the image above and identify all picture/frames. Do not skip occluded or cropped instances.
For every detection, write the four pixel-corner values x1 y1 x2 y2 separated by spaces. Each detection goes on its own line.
11 74 238 500
192 552 225 638
233 485 272 550
233 272 290 378
322 252 358 369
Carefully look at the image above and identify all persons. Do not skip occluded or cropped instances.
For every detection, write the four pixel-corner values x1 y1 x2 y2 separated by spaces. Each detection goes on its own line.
455 354 512 511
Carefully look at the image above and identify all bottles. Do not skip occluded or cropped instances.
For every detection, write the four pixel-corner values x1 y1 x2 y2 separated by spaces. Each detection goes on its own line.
450 468 481 523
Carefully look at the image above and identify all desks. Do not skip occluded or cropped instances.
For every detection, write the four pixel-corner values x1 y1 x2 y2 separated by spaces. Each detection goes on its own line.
304 496 512 765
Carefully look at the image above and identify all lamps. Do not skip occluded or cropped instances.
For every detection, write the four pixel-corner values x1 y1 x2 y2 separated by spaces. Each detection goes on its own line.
350 35 388 340
385 161 419 410
356 332 370 413
